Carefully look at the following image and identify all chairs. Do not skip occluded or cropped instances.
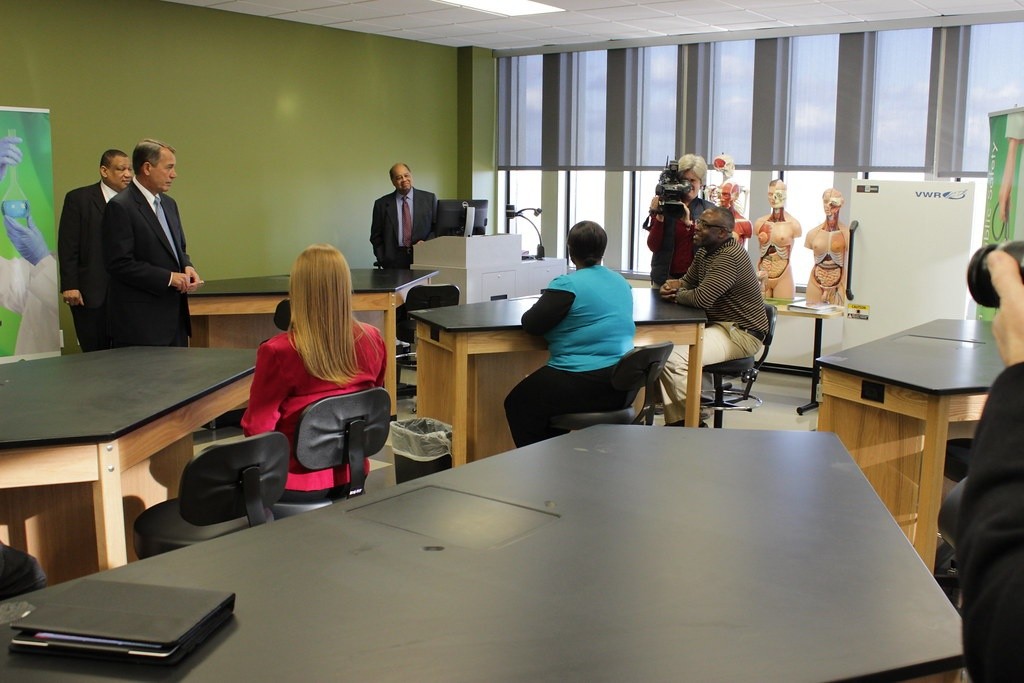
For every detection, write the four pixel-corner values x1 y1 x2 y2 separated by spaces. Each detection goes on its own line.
702 303 779 428
395 282 459 401
134 387 392 560
551 341 674 430
273 295 292 332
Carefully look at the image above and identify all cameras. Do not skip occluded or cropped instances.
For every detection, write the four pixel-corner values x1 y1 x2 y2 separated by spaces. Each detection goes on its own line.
968 241 1024 308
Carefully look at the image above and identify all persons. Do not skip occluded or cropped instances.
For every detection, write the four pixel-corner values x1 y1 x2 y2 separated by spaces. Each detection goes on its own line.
58 149 134 353
999 111 1024 240
659 206 769 426
104 138 200 349
369 163 438 270
953 239 1024 683
241 244 387 504
647 154 717 288
504 220 636 448
0 136 58 355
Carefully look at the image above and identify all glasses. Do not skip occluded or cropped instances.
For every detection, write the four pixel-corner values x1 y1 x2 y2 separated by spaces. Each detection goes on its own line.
694 219 733 233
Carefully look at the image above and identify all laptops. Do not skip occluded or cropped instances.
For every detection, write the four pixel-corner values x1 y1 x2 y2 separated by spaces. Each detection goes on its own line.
9 579 236 665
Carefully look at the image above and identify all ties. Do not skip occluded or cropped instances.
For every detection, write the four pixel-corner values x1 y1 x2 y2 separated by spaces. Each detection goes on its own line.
153 197 180 268
401 195 413 247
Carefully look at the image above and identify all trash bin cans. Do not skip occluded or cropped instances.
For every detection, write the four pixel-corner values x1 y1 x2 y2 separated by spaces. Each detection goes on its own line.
391 417 453 485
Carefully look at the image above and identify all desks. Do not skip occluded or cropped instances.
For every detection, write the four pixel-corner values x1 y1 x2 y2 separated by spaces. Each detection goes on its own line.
776 303 845 415
408 285 708 470
187 266 441 422
0 345 258 589
0 424 973 683
815 318 1007 576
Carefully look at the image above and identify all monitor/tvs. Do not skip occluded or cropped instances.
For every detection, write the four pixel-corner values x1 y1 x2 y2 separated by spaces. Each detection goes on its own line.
435 199 489 237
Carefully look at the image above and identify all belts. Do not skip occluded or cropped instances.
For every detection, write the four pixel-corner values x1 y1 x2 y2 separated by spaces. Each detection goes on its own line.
399 248 414 255
739 326 766 342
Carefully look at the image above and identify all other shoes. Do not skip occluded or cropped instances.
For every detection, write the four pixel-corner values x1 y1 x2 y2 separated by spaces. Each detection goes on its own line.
666 418 709 428
653 402 666 416
